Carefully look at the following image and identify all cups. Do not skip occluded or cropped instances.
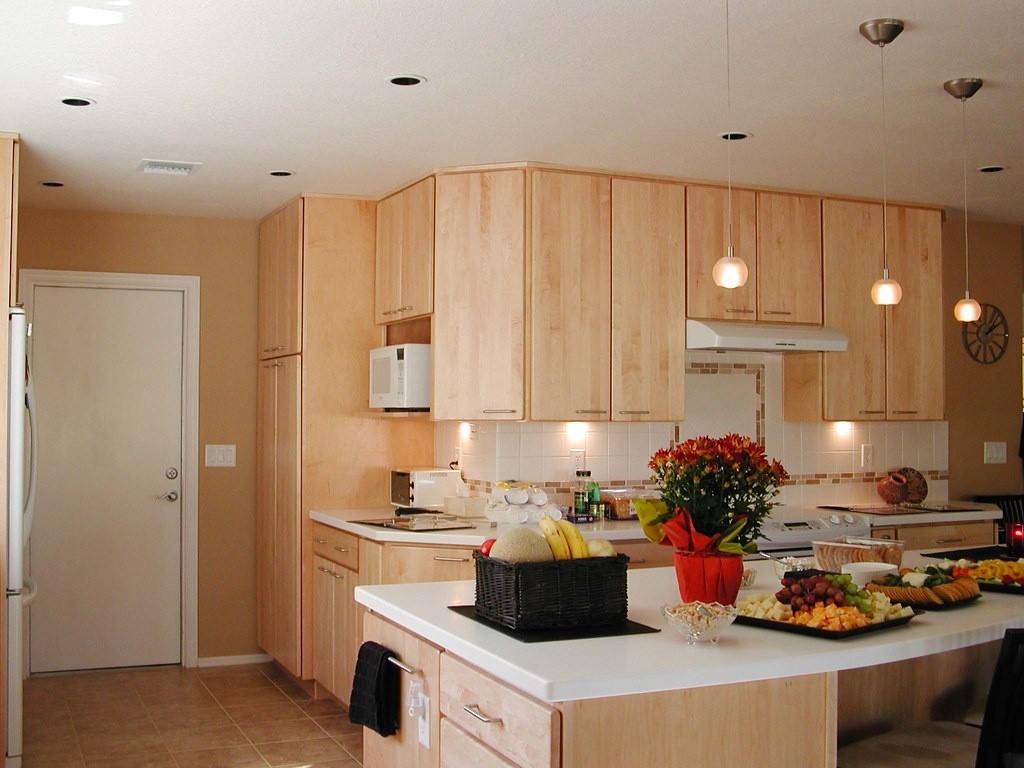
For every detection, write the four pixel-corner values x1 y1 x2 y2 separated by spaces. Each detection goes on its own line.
486 487 562 524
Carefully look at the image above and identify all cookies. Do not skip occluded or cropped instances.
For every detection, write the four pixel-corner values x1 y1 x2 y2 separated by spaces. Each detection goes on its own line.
818 542 902 573
865 568 979 604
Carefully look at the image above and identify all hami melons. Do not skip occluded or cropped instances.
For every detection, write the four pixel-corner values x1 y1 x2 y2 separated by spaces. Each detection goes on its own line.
489 527 554 562
789 602 868 630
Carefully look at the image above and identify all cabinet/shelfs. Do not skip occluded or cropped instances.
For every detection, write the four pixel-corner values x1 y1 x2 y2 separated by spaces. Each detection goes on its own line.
783 198 945 423
686 185 822 325
363 610 443 768
258 193 387 360
435 169 525 421
383 542 482 586
608 538 674 570
256 355 435 702
530 171 685 422
310 507 384 716
375 172 434 325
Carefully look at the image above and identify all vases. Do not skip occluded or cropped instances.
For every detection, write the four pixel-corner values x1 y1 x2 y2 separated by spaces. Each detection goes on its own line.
674 551 743 606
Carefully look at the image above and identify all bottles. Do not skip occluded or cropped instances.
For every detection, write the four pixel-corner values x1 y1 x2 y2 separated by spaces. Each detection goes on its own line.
598 501 611 520
574 471 600 514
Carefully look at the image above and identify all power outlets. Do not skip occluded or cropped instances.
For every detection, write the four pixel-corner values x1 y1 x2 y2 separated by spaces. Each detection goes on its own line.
570 450 585 481
861 444 872 468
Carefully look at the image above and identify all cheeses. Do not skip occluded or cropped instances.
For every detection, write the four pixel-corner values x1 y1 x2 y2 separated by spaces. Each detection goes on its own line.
864 589 915 622
735 594 793 620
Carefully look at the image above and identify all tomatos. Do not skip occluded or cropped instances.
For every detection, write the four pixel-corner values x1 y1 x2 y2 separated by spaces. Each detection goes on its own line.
481 538 496 556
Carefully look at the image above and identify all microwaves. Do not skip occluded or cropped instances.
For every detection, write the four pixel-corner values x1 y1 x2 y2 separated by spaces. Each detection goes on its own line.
369 344 430 407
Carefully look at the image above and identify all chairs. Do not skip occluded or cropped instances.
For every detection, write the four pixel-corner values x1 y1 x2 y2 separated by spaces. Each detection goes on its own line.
836 629 1024 768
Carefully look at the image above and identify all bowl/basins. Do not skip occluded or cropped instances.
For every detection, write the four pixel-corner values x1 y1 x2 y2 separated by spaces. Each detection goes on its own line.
661 600 737 645
774 556 815 580
841 562 899 586
739 568 758 589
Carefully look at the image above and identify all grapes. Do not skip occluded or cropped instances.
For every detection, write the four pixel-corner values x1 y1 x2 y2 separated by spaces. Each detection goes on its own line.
776 575 872 613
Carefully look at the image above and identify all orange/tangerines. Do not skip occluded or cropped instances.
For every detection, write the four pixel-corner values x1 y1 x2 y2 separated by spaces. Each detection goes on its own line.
973 559 1024 580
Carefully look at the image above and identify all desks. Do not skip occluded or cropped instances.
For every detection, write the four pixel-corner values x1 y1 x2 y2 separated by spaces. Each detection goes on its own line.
356 543 1024 768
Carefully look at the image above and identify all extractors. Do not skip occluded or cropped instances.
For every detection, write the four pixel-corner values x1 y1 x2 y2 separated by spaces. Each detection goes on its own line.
685 318 848 352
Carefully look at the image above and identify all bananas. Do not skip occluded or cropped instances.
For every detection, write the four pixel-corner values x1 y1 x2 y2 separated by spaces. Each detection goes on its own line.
539 516 590 560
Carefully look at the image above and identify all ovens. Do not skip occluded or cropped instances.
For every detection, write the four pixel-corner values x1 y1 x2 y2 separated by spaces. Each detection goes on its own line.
390 468 461 508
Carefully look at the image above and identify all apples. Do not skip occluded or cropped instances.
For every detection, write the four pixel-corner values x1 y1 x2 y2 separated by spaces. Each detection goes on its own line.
586 539 617 556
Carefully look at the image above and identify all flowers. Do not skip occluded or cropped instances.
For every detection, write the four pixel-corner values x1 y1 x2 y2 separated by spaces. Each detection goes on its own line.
648 433 790 553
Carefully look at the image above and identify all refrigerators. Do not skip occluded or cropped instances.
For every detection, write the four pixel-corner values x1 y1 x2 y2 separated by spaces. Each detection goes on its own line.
0 306 40 768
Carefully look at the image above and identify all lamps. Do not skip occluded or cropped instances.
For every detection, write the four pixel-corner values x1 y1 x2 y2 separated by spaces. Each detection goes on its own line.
860 18 903 305
711 0 748 287
944 78 982 321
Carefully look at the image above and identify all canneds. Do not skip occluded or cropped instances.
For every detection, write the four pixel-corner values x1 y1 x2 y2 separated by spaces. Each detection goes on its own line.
574 491 610 521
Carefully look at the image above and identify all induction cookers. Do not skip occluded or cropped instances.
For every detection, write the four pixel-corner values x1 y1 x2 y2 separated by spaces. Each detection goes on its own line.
728 499 870 552
